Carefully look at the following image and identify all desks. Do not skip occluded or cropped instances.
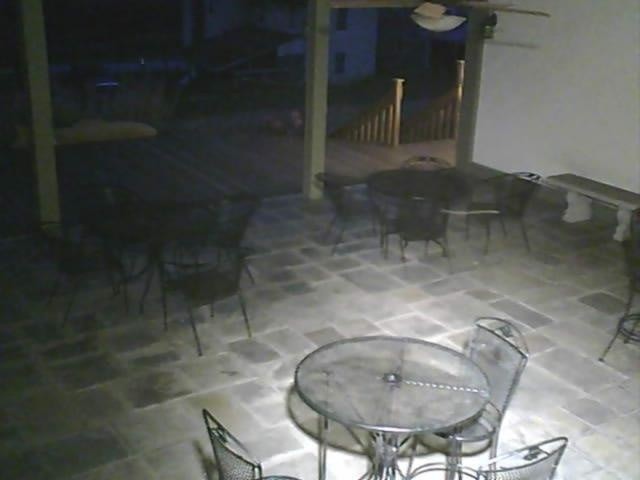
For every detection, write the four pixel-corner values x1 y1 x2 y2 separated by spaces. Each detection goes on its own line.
295 336 491 480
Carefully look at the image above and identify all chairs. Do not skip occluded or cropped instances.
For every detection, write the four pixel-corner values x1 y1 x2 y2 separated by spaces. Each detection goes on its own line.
37 185 259 356
476 436 569 480
599 207 640 363
316 156 542 265
405 316 530 480
202 408 301 480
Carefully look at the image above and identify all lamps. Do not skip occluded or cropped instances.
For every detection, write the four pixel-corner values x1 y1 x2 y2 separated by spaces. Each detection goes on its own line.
411 2 467 33
